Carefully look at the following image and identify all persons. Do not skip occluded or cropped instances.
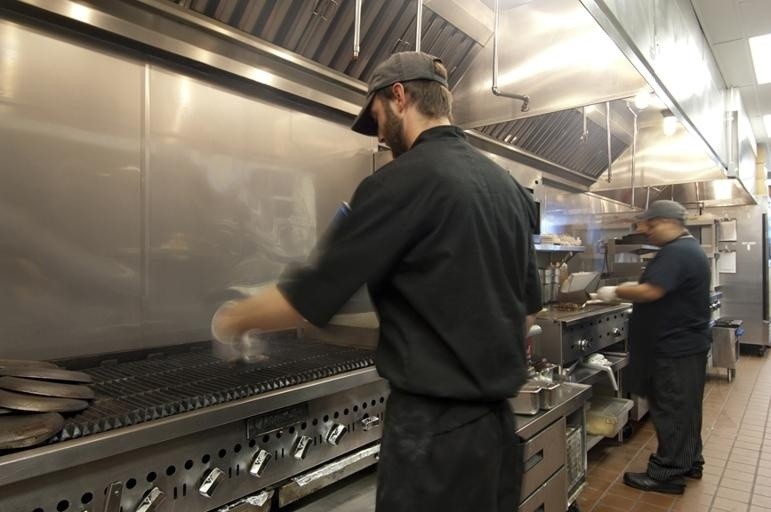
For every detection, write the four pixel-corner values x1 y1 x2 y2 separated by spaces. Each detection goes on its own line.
211 49 543 511
597 200 712 495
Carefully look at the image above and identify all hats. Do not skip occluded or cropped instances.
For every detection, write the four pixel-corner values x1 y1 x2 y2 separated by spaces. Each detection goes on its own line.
351 51 449 137
632 200 688 222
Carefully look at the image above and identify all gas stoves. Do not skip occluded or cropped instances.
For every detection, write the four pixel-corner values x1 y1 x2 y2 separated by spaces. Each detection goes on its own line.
531 301 635 368
0 324 392 509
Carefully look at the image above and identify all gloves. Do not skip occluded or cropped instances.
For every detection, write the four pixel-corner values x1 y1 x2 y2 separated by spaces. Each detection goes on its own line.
595 285 624 306
210 299 252 363
619 281 639 287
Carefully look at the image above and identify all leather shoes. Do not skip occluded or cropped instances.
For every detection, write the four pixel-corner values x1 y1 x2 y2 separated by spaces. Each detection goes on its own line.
623 471 685 495
648 452 704 480
